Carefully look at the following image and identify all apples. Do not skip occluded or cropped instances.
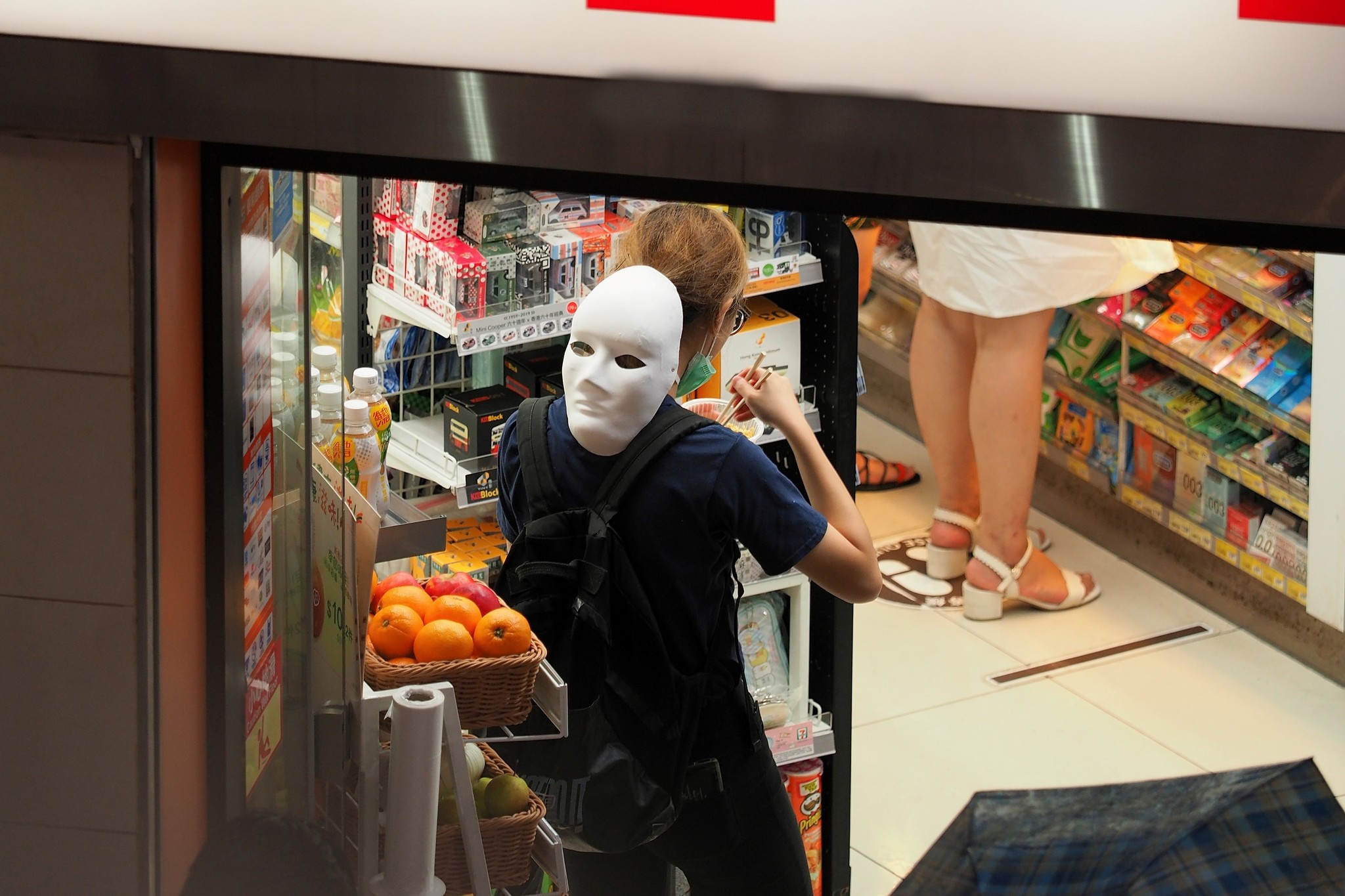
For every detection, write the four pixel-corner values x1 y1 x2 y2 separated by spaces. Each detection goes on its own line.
425 571 474 600
449 583 501 617
370 571 425 615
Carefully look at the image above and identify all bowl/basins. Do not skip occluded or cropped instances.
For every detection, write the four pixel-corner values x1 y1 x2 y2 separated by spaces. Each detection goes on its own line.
681 397 765 446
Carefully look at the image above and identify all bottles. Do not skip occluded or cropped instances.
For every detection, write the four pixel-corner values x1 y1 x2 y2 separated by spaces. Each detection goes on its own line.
268 332 396 526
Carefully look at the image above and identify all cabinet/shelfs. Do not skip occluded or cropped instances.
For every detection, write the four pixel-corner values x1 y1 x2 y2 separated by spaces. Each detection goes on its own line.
859 211 1318 673
365 242 839 791
363 652 575 896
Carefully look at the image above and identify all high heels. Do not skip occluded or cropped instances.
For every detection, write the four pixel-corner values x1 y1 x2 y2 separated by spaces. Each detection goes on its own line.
962 536 1101 620
925 505 1051 579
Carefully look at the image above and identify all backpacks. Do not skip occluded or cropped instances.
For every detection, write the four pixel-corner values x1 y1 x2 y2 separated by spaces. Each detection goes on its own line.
488 396 745 855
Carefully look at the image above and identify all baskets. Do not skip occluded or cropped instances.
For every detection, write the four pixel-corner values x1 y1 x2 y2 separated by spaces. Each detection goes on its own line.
323 732 546 896
364 574 547 729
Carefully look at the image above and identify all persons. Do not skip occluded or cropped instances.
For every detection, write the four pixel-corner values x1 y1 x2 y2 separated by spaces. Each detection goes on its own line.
843 214 922 492
497 201 883 895
907 220 1184 622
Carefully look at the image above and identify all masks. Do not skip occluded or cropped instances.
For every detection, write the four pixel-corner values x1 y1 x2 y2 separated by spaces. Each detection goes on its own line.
676 314 726 398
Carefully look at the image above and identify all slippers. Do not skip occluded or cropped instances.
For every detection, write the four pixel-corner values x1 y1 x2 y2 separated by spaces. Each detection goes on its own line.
856 449 921 492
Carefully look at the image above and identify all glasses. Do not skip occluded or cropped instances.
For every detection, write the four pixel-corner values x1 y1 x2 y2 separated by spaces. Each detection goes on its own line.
730 304 751 336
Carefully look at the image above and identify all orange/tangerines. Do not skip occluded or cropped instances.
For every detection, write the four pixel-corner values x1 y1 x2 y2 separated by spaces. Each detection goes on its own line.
366 570 532 665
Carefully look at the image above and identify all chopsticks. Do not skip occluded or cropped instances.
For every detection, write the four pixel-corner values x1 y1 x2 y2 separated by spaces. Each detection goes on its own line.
715 352 773 427
